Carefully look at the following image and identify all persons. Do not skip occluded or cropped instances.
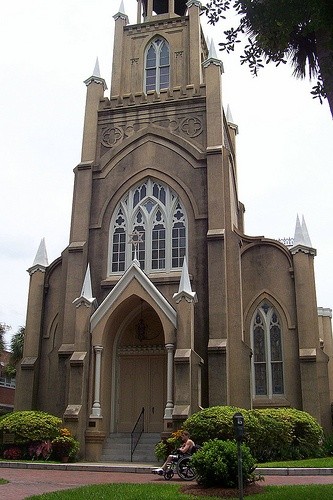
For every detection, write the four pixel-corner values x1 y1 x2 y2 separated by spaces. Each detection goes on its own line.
160 430 195 471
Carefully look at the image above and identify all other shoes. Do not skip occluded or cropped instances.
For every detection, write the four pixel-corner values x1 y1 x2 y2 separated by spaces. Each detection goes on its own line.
152 468 163 474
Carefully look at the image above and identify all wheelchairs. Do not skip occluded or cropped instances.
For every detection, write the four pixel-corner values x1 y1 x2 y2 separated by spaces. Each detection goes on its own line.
152 445 201 479
